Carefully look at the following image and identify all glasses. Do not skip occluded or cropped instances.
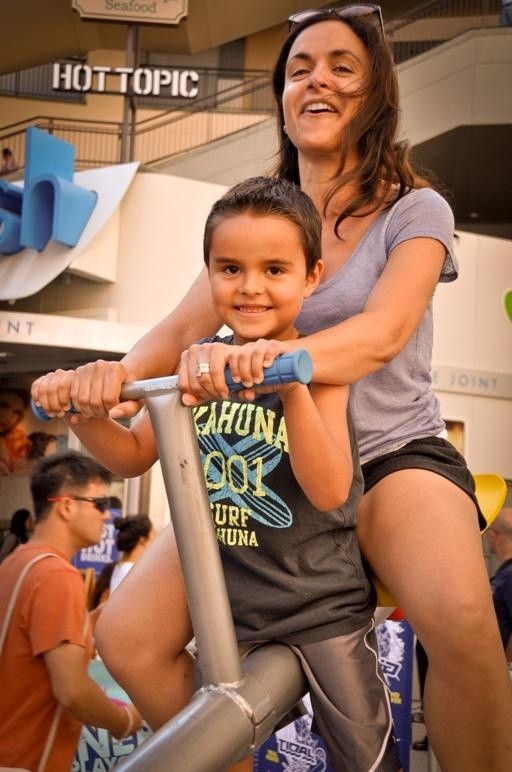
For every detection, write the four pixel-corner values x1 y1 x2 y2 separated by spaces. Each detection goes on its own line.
284 2 392 67
44 485 113 513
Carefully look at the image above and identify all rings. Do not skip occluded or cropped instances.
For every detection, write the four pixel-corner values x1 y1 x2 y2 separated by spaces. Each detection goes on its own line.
195 362 210 377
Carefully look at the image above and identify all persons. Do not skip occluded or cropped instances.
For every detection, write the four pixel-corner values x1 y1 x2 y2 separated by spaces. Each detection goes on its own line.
1 430 156 772
70 7 512 771
412 508 512 751
30 174 407 772
